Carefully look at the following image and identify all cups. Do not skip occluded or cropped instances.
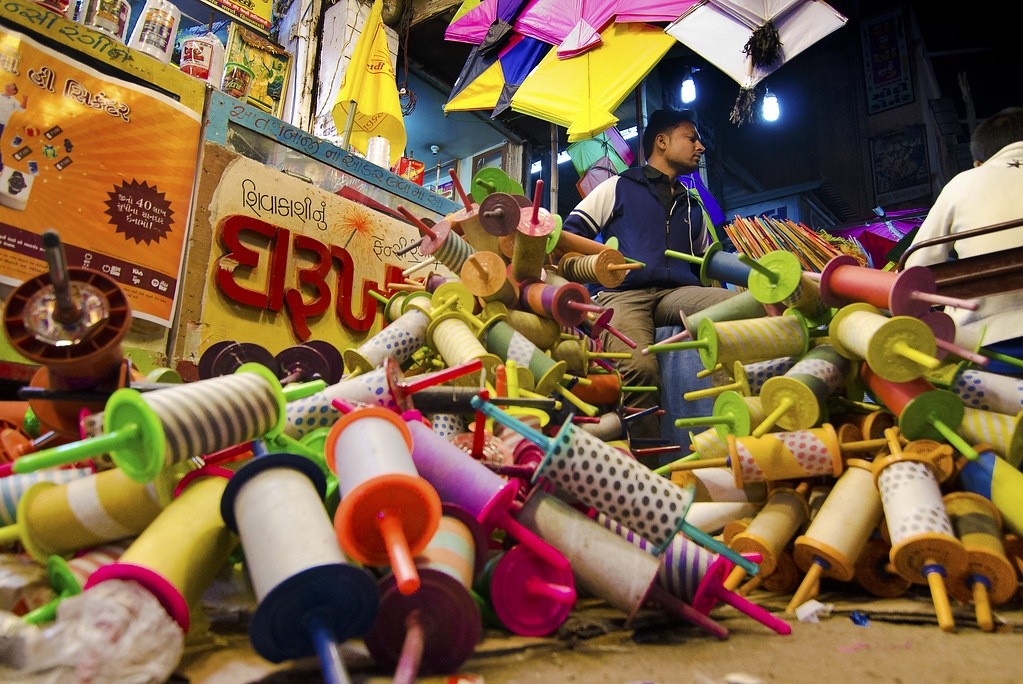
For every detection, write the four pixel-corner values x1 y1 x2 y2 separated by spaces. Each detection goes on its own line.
66 0 181 66
220 61 256 103
366 137 390 170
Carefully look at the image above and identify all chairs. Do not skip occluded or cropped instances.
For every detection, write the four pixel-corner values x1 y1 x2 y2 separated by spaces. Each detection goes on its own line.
894 217 1023 308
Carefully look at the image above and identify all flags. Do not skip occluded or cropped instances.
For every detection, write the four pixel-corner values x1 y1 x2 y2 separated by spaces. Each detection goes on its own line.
332 0 407 167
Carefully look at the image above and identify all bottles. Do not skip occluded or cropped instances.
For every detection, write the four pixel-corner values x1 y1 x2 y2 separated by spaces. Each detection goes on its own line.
179 32 225 88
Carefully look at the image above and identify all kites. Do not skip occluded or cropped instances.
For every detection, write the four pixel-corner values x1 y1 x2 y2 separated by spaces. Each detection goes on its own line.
441 0 849 226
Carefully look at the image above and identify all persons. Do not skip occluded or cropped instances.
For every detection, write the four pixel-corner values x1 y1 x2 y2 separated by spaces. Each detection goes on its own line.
563 105 742 444
904 107 1023 380
